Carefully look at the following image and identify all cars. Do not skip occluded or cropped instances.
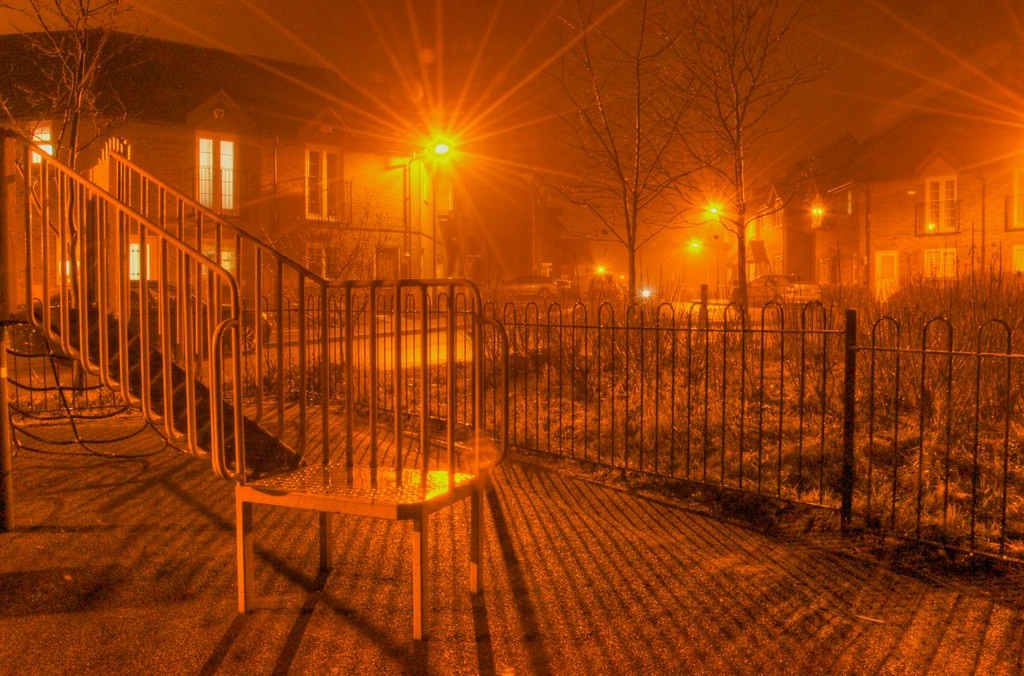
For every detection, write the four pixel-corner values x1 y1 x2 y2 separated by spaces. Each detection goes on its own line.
498 274 558 301
7 278 272 367
728 274 823 309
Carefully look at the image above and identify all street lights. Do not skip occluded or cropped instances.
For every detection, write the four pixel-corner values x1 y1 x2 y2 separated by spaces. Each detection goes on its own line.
690 241 720 295
408 143 451 319
